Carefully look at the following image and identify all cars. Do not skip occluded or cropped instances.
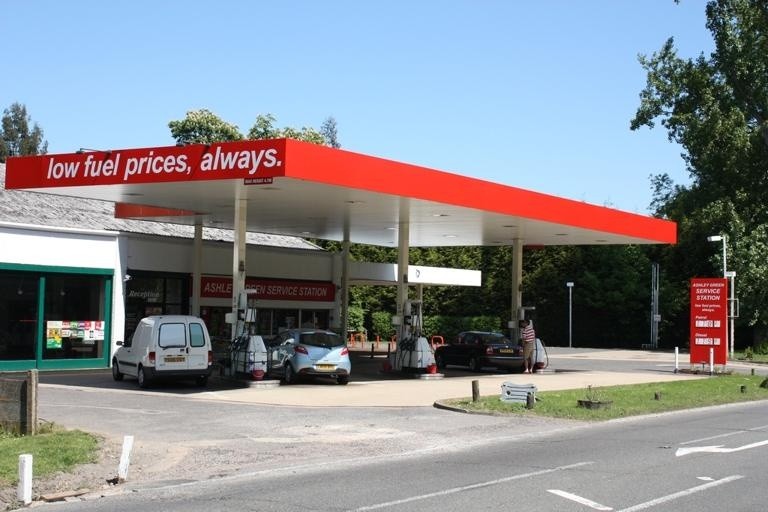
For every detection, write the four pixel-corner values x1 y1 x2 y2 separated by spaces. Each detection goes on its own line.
435 332 526 374
266 328 351 386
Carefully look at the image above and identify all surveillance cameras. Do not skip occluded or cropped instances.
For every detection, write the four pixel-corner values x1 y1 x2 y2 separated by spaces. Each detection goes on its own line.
124 275 132 280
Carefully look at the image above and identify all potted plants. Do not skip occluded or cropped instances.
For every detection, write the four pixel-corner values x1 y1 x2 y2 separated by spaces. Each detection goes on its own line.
576 384 614 411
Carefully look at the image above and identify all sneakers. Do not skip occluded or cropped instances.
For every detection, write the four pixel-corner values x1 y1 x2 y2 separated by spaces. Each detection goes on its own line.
524 368 532 373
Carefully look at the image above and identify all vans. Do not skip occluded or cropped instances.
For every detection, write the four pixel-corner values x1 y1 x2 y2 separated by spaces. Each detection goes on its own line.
109 315 215 387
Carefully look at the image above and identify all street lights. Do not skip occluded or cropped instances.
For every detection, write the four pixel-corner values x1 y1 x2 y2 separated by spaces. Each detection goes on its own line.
706 235 726 280
566 282 573 347
726 271 735 360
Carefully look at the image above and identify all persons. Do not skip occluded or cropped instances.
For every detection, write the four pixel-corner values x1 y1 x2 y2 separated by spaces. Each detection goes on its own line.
520 320 535 373
